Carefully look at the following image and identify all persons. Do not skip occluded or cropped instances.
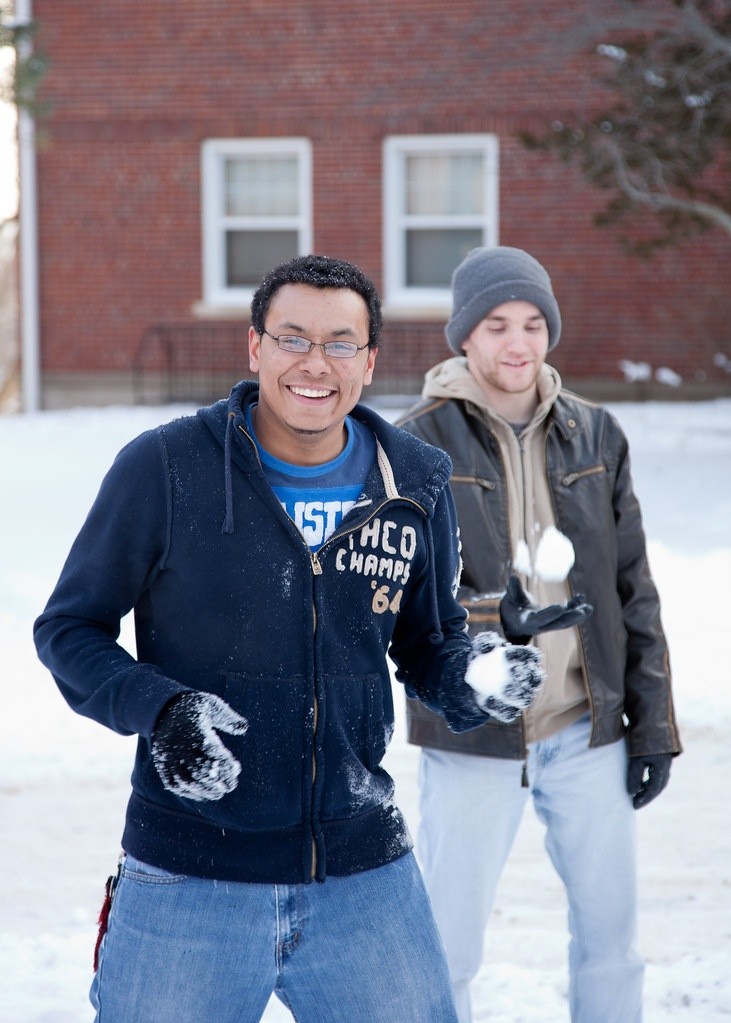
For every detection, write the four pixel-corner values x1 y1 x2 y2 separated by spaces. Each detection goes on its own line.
384 241 684 1020
32 256 543 1023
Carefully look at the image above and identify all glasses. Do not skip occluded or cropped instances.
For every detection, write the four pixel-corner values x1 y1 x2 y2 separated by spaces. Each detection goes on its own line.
263 329 370 358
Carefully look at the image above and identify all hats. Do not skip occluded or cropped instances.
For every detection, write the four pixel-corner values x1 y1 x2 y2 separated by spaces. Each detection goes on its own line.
441 245 562 357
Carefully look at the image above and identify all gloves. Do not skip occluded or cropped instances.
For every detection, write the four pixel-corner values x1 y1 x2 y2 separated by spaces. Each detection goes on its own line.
150 691 249 804
497 574 593 637
468 631 543 724
626 752 673 808
